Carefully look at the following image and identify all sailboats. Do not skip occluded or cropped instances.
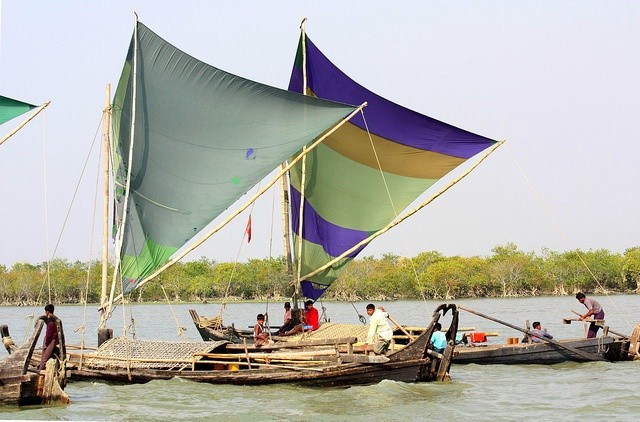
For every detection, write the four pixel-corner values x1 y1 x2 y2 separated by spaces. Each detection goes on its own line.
1 95 73 408
188 17 640 363
0 11 459 385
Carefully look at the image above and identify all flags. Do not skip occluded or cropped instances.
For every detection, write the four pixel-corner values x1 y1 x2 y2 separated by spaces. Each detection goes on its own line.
246 215 251 243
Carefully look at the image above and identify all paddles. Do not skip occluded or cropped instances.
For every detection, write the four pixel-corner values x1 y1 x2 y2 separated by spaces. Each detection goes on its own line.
457 305 608 361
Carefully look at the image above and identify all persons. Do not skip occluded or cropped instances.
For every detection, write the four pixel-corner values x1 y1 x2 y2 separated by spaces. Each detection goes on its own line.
253 314 268 346
279 300 319 336
362 304 393 355
575 292 604 338
428 323 448 354
529 322 553 343
284 301 291 325
33 304 64 370
